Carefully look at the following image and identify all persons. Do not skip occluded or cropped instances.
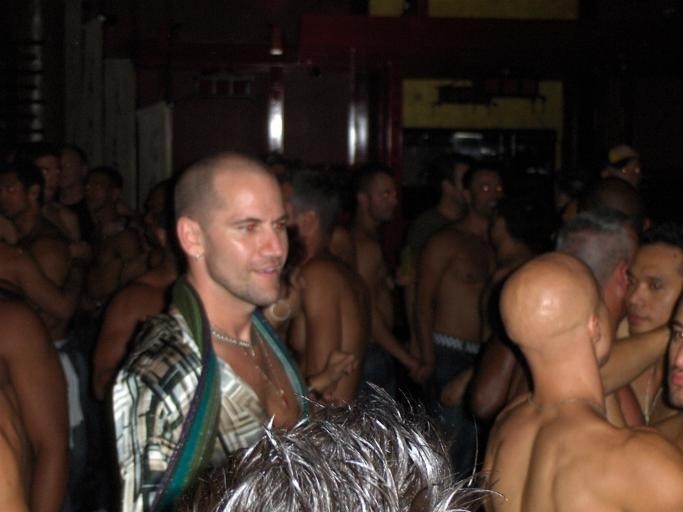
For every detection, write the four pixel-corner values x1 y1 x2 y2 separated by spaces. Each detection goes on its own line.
2 125 682 512
